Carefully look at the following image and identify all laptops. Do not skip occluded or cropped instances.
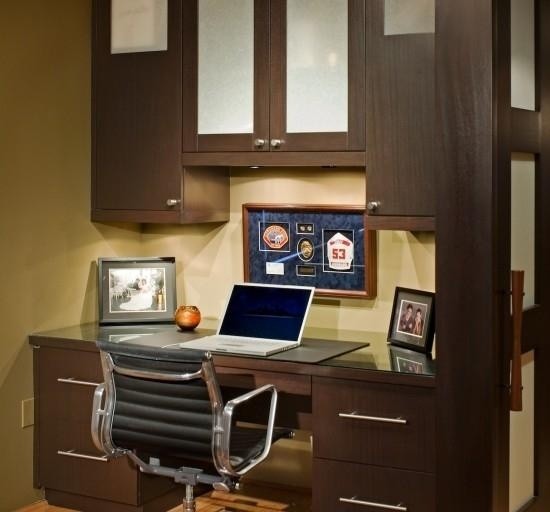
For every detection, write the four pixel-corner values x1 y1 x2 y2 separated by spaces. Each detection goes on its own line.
180 281 315 357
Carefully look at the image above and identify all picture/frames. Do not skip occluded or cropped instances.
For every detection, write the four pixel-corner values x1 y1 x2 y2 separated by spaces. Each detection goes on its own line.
387 286 436 354
242 202 377 300
102 326 166 344
389 345 434 375
98 257 177 323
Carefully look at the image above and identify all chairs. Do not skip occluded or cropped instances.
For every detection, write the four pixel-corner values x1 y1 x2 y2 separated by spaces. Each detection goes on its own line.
91 341 295 512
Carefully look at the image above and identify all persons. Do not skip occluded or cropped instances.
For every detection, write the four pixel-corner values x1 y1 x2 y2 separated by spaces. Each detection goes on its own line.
411 308 424 335
400 304 414 333
119 279 153 311
133 278 141 290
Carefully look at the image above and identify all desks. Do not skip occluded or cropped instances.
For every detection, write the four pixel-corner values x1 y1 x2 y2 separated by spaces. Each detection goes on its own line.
29 317 436 512
